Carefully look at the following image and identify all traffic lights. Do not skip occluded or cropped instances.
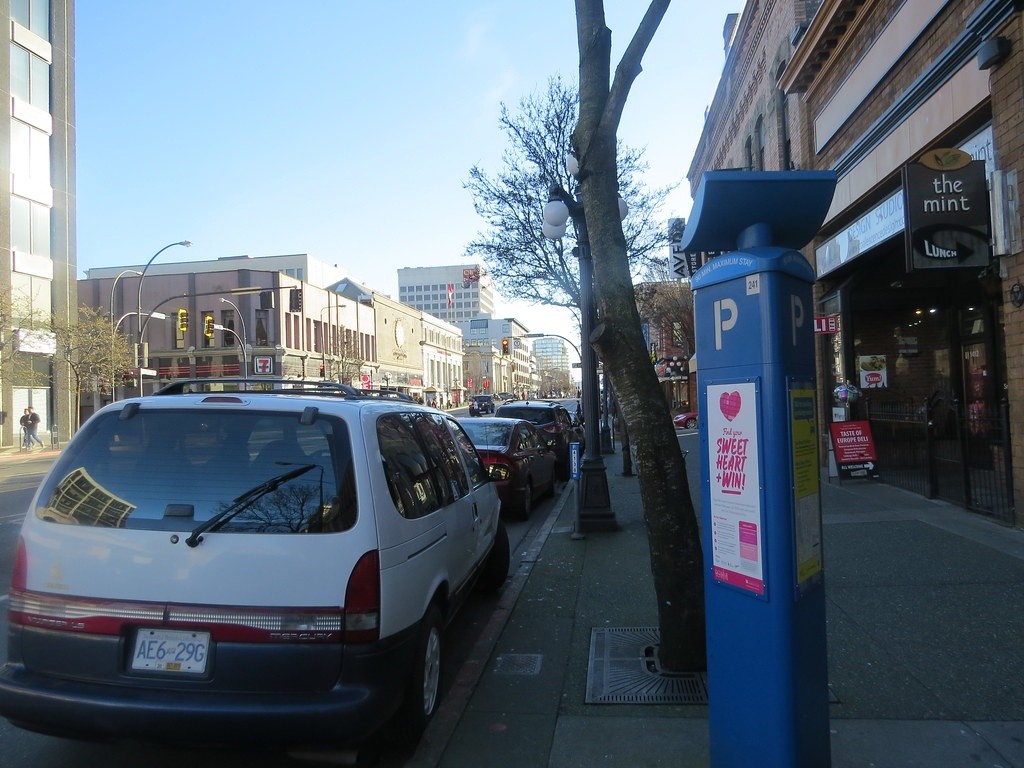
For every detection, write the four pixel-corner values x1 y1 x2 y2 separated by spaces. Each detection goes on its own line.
320 366 325 378
502 338 510 355
204 316 215 337
178 308 189 332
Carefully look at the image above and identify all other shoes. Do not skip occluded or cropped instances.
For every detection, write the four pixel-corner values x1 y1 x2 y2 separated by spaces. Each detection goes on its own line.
33 443 37 448
41 441 45 449
22 446 26 449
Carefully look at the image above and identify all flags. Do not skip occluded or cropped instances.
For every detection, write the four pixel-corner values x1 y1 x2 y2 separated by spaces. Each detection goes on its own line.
449 283 453 305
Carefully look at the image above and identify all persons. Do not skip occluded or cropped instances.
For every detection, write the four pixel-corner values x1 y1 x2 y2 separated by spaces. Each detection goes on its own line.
469 397 483 417
448 400 452 408
522 392 525 400
20 407 44 450
415 397 438 408
576 401 580 416
541 393 547 399
269 427 307 458
208 437 251 470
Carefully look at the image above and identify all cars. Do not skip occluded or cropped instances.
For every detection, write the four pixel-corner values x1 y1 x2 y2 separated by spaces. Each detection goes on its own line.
454 418 556 522
469 395 495 415
498 392 513 400
493 399 585 480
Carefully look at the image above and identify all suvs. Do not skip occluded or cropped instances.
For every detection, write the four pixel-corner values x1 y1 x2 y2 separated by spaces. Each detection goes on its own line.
0 379 513 755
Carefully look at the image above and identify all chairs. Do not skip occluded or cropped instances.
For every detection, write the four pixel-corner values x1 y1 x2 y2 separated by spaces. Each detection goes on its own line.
134 432 333 511
463 428 477 443
492 430 505 445
514 412 523 418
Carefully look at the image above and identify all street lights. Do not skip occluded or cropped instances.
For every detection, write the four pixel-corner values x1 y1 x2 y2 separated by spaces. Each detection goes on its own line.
542 151 632 545
111 269 143 403
137 240 193 343
321 303 347 379
218 297 248 381
